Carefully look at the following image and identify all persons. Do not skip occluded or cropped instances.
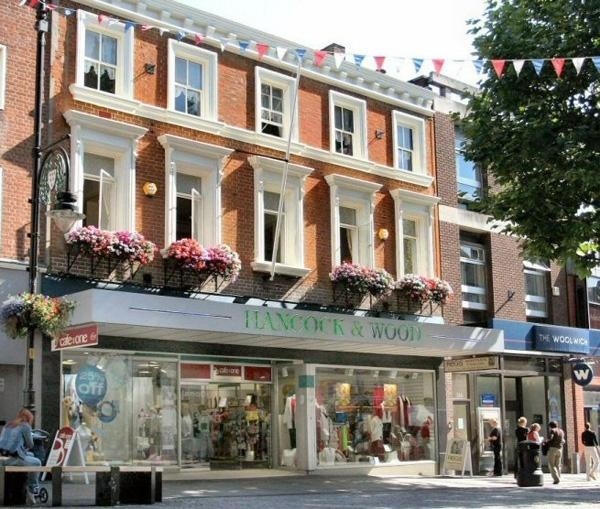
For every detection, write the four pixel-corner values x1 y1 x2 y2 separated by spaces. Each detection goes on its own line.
582 422 599 482
396 394 412 428
528 422 544 450
0 408 42 505
283 394 298 449
545 420 565 485
486 417 502 477
516 417 530 473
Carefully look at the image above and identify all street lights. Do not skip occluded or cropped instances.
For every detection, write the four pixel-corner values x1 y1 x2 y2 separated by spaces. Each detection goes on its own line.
21 146 86 429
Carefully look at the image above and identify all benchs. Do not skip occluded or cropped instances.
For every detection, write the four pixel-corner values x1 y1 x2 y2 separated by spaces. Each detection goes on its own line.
0 464 164 507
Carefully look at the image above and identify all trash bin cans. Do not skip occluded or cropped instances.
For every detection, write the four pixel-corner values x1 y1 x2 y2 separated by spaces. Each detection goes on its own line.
517 440 545 487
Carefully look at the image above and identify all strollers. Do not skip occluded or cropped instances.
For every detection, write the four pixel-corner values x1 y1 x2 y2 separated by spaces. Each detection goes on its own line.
1 420 49 503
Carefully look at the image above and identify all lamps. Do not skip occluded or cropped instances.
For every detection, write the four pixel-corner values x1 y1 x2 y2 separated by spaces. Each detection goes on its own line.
142 180 158 197
378 228 389 241
